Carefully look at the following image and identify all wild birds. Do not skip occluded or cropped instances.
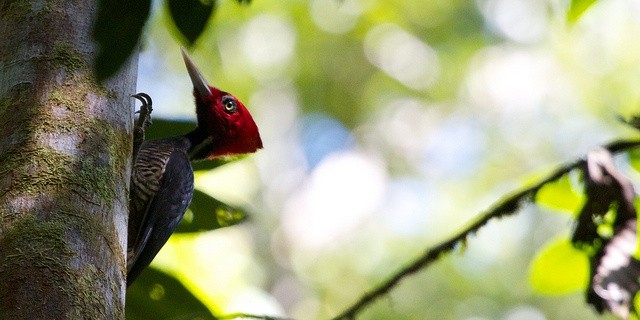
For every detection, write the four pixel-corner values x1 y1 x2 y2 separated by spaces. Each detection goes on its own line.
124 48 264 283
